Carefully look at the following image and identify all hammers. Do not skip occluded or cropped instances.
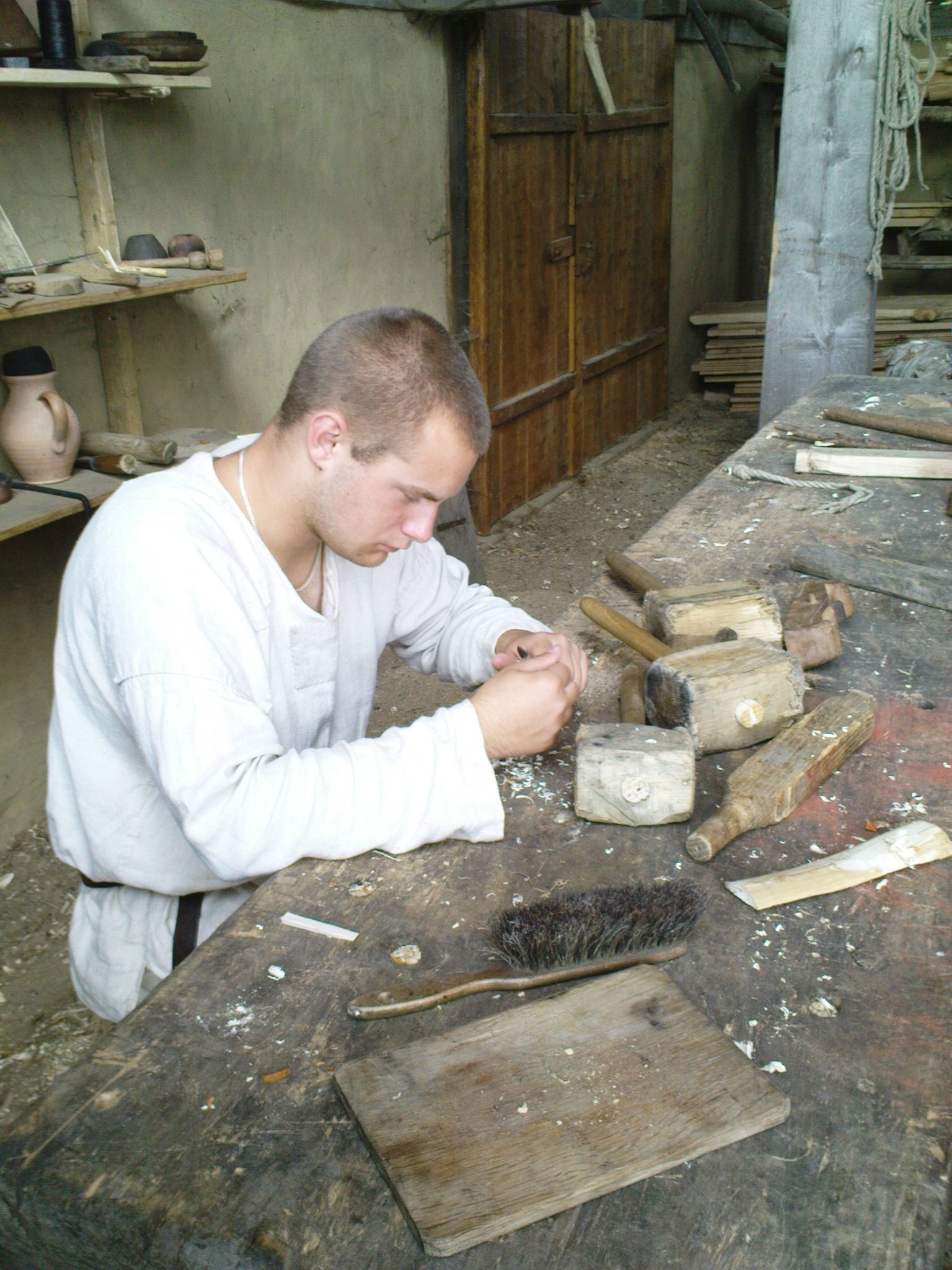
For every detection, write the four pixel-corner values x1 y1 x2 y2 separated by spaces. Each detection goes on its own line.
577 595 804 755
603 546 783 647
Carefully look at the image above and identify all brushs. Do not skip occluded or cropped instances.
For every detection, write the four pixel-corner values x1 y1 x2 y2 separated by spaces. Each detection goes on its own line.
343 880 708 1021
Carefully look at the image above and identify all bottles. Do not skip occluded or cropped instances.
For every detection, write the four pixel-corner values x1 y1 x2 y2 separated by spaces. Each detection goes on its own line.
0 369 80 485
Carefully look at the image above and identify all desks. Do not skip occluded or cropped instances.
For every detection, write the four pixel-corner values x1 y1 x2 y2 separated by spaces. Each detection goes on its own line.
0 370 952 1270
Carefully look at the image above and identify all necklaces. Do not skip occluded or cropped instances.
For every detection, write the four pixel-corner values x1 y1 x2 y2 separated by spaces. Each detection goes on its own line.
238 447 322 592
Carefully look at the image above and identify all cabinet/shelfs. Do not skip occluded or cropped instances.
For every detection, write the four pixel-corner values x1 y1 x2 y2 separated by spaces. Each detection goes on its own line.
0 51 248 542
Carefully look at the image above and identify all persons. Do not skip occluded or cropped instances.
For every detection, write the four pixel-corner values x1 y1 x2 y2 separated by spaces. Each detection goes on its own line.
44 304 592 1023
